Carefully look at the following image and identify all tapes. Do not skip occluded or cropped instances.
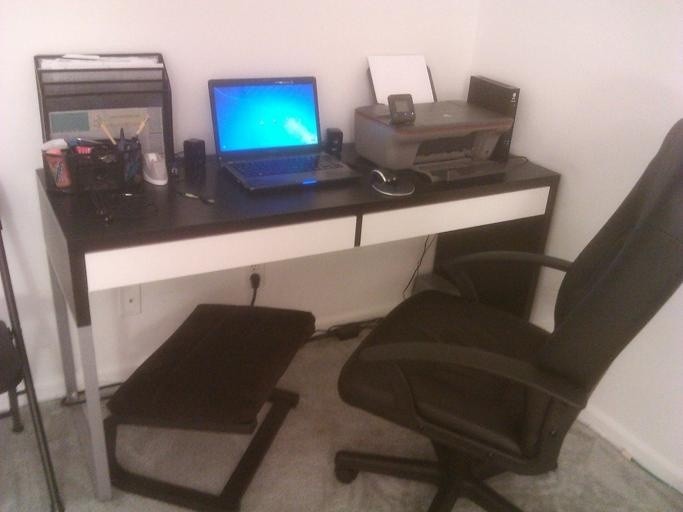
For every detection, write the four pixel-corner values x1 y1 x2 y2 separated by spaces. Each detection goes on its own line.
145 153 157 164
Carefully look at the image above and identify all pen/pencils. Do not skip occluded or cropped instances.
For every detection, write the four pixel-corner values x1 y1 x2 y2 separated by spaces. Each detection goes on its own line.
176 190 215 204
76 112 150 152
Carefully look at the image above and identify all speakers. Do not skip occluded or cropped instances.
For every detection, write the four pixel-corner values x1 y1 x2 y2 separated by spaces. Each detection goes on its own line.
326 127 343 154
183 137 206 165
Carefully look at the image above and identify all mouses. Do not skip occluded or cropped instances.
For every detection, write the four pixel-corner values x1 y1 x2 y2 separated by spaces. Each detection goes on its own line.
368 167 395 186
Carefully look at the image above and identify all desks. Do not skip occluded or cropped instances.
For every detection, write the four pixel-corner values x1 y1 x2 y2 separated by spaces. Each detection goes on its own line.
36 141 560 500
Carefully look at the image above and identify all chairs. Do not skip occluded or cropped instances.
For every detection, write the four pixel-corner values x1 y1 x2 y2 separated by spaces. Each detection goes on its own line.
334 124 683 512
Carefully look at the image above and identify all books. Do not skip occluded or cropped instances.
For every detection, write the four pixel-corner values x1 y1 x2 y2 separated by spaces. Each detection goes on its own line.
36 55 165 112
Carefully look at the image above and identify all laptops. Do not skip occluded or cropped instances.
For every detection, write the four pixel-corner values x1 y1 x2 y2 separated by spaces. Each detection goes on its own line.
208 76 362 193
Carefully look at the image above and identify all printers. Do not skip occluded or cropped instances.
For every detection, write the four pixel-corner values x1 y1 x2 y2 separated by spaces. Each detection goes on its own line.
353 64 515 184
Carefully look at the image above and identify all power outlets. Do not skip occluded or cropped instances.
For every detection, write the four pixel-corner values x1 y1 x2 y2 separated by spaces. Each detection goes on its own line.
244 262 267 292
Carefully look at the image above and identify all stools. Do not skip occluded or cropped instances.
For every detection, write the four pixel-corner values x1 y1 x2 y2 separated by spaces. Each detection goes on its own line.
105 302 316 509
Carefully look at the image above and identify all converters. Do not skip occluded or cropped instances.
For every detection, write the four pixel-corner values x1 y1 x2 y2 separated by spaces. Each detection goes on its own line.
334 324 362 340
